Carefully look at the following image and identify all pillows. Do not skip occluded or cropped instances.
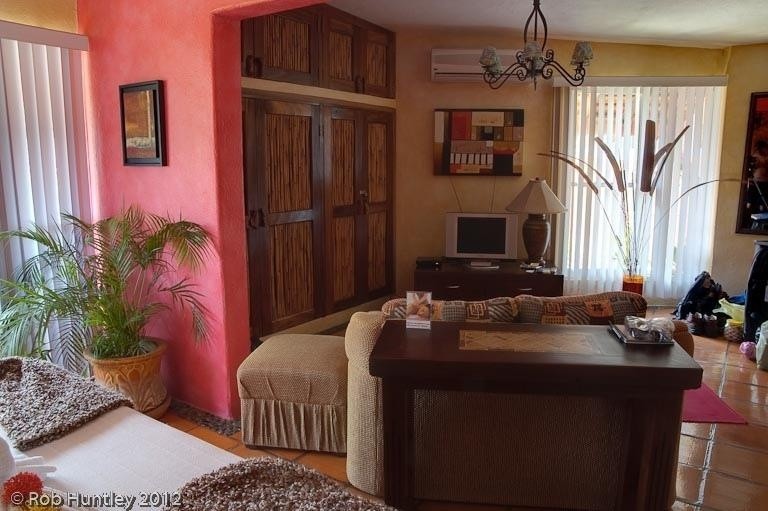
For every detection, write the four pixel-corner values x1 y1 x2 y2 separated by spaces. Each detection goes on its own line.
390 297 518 323
516 292 648 325
0 425 55 507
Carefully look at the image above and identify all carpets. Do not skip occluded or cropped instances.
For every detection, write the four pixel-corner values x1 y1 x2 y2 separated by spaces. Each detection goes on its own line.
682 381 748 426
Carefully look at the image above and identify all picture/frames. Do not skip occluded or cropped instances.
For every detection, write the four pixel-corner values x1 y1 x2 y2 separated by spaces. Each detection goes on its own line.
119 80 166 166
735 91 768 236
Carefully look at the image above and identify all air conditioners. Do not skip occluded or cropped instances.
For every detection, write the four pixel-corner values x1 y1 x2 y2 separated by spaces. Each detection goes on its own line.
430 49 530 86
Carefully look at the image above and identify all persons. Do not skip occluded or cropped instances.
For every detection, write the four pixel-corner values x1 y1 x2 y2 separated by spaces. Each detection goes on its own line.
408 303 430 318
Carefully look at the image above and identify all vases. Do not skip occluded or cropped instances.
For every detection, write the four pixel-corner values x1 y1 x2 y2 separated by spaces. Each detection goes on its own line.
621 272 644 295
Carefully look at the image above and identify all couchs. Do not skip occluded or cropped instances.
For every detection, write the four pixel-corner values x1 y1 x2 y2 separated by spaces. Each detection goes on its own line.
1 356 396 510
346 311 697 497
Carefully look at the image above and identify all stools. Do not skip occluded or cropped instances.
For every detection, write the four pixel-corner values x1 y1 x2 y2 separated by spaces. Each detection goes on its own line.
238 334 348 454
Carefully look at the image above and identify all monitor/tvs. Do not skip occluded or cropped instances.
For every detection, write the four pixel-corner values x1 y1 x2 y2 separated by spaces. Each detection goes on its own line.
443 212 519 267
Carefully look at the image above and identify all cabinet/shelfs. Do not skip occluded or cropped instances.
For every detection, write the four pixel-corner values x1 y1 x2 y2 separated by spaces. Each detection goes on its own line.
370 319 704 511
321 105 396 315
243 98 320 337
240 3 319 83
321 1 398 99
414 257 564 302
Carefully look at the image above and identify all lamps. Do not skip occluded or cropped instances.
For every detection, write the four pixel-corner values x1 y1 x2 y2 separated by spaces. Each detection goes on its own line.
479 0 593 92
506 176 566 266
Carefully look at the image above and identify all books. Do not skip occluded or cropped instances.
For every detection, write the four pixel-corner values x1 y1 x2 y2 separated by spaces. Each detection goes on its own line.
613 324 675 345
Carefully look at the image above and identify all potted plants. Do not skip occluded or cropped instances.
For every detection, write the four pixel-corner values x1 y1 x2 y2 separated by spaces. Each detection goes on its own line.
0 196 218 420
